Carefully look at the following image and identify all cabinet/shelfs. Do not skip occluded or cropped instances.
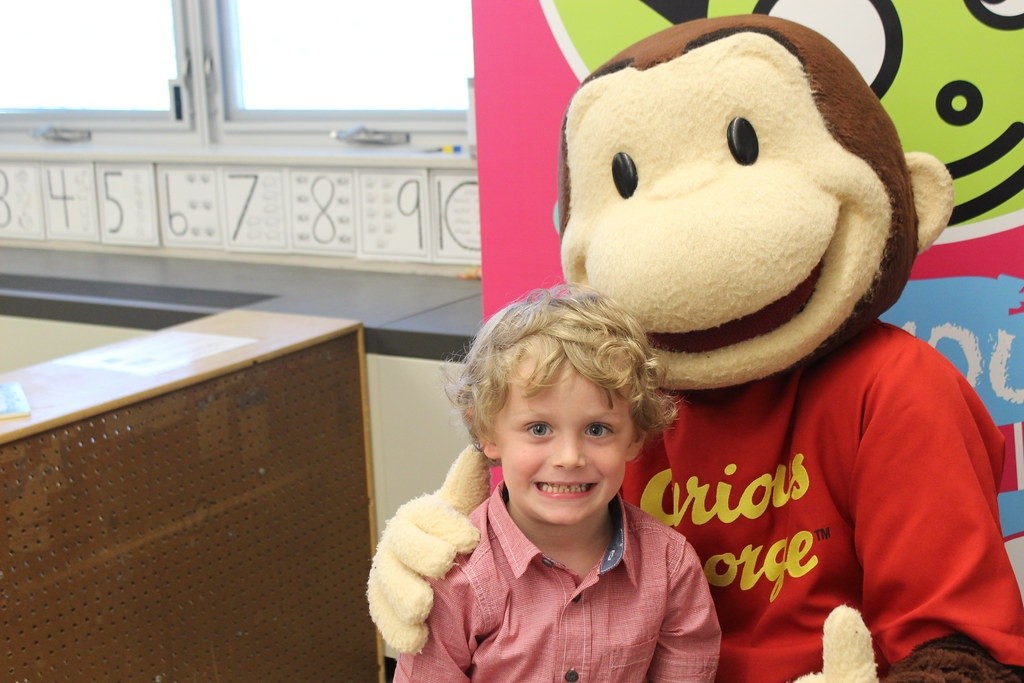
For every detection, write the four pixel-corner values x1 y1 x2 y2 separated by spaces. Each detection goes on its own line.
0 307 384 681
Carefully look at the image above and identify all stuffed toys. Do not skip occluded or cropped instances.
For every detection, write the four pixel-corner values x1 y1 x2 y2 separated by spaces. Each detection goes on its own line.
368 14 1024 682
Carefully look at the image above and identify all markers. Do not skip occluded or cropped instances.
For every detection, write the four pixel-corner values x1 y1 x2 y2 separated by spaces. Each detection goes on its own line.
43 132 91 141
344 133 409 144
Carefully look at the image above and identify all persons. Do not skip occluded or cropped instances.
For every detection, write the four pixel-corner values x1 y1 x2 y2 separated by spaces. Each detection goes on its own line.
393 283 726 682
